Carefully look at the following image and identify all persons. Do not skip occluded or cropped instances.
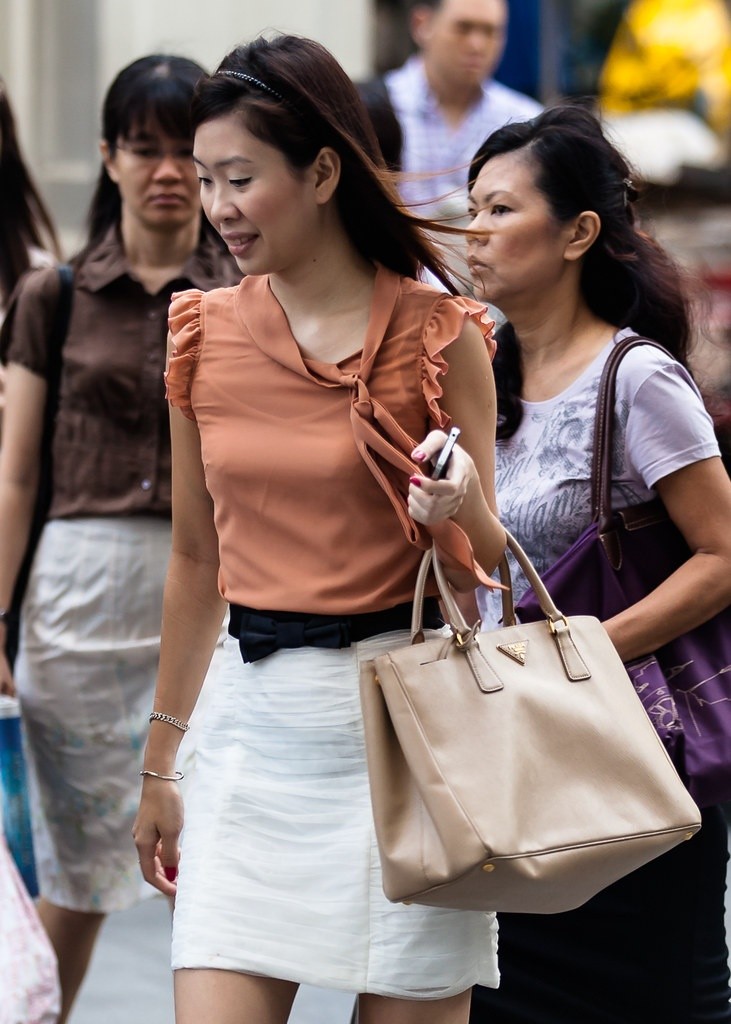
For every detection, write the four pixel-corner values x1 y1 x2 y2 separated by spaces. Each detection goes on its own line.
465 106 731 1024
131 33 507 1022
351 0 547 338
0 54 246 1023
0 73 65 423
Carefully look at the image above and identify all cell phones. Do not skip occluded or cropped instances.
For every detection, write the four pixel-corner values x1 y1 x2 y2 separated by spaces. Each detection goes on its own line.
431 427 461 480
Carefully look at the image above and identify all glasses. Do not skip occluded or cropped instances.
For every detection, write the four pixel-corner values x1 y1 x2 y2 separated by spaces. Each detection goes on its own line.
112 144 193 161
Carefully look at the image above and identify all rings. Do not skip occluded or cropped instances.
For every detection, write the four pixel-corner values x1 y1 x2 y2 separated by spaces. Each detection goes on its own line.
137 858 141 863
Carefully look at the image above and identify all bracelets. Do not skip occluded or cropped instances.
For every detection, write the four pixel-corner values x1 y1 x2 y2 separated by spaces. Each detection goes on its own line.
139 768 184 781
147 710 191 733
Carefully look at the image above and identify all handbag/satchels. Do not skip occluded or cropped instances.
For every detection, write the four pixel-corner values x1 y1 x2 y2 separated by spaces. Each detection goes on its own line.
513 336 731 796
357 524 701 914
0 696 61 1024
